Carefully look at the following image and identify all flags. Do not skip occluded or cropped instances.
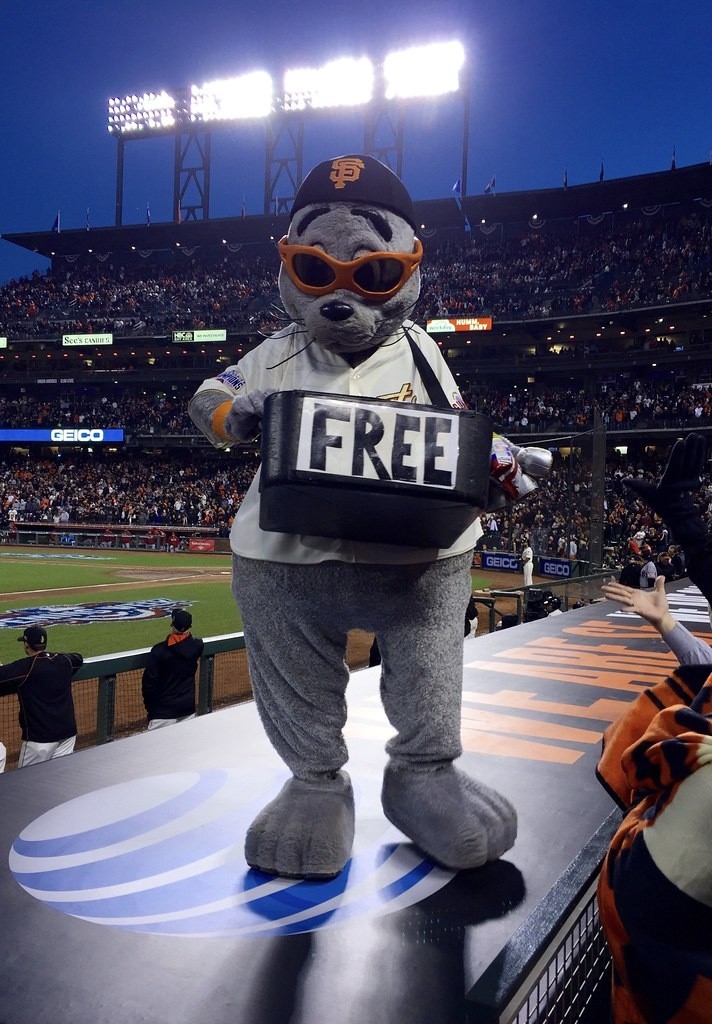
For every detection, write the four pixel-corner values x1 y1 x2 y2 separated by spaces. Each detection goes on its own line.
450 179 461 192
484 177 495 192
563 150 676 191
51 214 58 231
85 197 279 231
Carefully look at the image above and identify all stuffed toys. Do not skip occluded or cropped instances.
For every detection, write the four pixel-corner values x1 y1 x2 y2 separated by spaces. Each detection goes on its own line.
595 664 712 1024
186 154 553 881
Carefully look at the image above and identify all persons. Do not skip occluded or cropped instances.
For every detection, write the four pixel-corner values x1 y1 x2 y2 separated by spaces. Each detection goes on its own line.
140 608 204 731
601 575 712 664
0 627 83 769
639 553 658 590
522 541 534 586
0 225 712 640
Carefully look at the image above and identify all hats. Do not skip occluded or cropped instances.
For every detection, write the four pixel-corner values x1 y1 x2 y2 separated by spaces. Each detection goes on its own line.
171 607 192 633
16 626 47 650
